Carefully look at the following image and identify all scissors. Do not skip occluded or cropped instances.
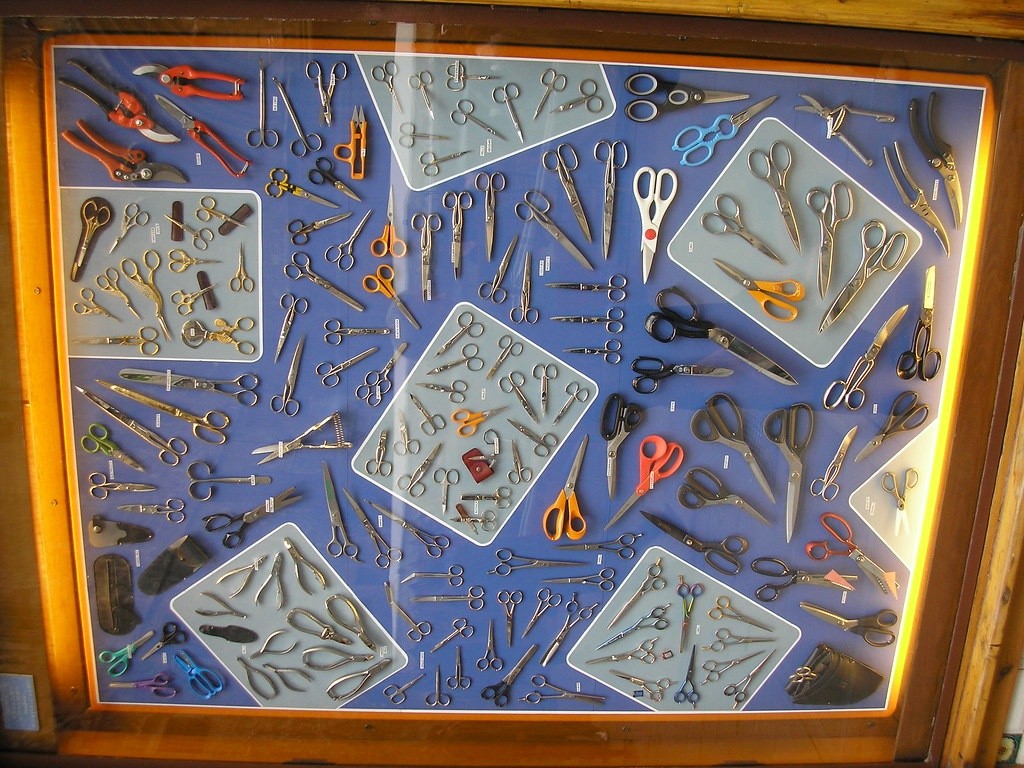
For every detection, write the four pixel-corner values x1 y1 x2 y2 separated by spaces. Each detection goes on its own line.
56 56 989 723
